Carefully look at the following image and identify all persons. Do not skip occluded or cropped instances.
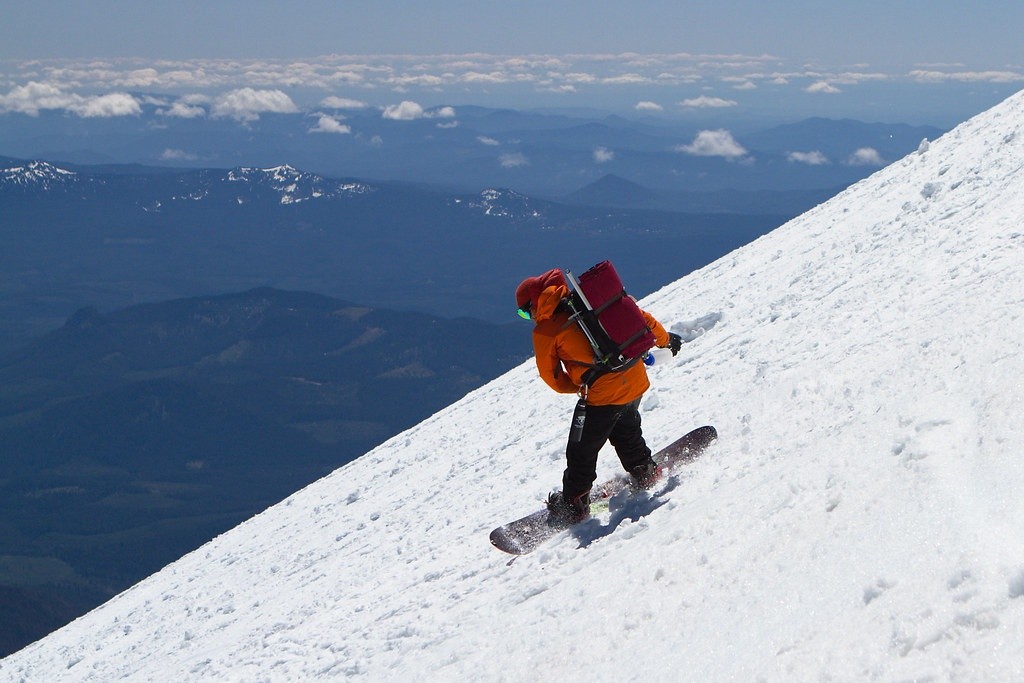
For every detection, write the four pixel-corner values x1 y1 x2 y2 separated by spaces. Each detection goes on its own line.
516 262 682 516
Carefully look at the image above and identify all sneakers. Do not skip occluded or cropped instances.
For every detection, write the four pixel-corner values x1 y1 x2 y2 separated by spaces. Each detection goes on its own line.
625 468 664 492
547 491 591 528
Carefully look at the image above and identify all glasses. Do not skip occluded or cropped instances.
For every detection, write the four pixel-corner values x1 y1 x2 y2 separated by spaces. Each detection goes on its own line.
516 297 534 321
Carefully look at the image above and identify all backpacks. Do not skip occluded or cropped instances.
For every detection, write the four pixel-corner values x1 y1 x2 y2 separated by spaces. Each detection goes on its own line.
568 260 658 363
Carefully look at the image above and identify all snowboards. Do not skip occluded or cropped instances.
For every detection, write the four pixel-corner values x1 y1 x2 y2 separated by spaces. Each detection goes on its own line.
488 425 719 557
